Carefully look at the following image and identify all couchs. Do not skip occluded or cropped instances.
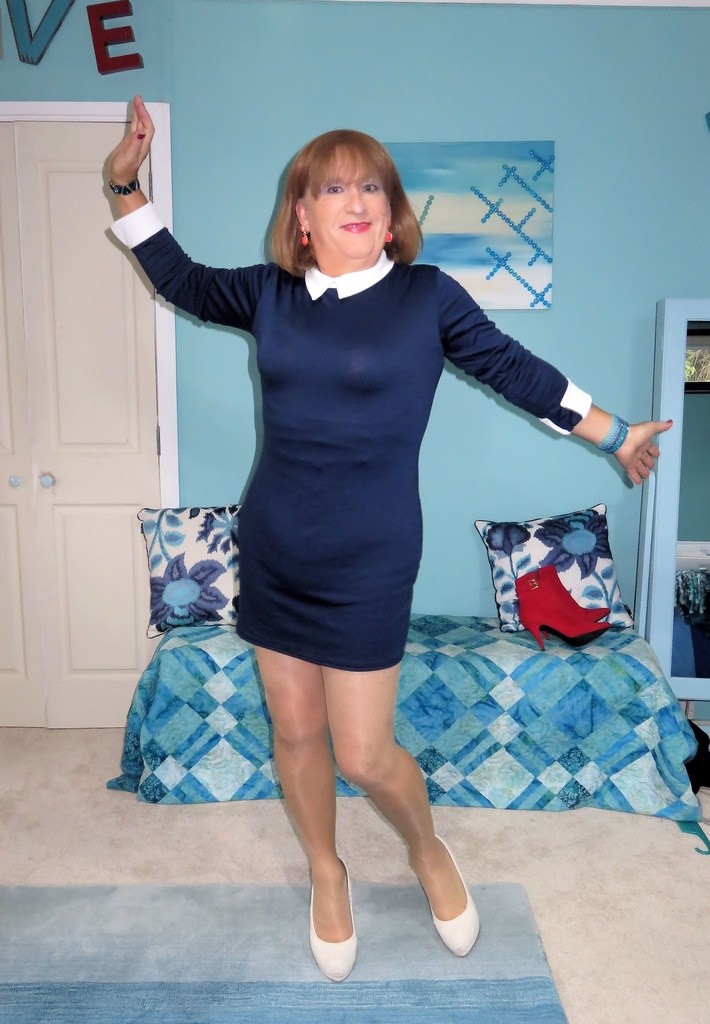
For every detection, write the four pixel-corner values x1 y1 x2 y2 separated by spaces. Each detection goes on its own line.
105 613 704 822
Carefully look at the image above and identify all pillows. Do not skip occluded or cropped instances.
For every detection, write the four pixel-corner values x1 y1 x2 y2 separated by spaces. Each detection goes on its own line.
474 502 635 633
136 505 242 640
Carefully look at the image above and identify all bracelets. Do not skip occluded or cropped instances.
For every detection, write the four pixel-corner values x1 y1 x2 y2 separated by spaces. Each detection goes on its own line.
596 415 628 454
109 178 141 195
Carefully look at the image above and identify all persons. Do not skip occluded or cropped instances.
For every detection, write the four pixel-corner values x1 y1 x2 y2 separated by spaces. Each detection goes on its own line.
108 95 673 983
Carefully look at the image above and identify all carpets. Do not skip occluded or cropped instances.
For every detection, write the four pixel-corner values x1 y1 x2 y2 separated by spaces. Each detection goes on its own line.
0 882 569 1024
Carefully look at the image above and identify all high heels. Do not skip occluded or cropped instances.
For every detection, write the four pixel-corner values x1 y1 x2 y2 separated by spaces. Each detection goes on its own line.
414 834 480 957
515 566 611 652
537 566 613 641
308 854 358 983
